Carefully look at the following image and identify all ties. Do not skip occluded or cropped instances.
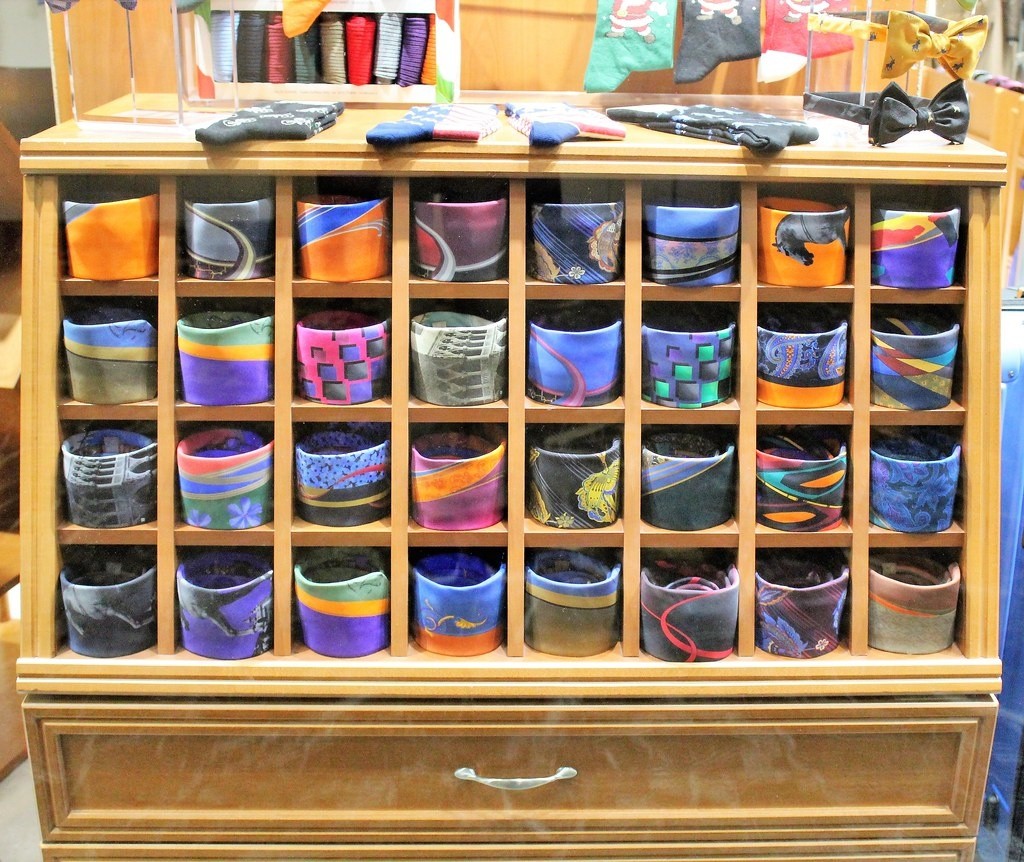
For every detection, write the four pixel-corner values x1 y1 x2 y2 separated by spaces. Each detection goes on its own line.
176 311 274 406
868 554 960 655
528 201 625 285
63 193 159 281
757 197 850 288
757 319 847 408
755 548 850 658
177 429 274 530
756 436 847 532
62 430 157 529
870 431 961 533
524 550 623 658
296 311 392 405
413 551 507 657
642 321 736 409
410 308 508 405
176 552 274 660
642 433 735 531
640 557 739 662
526 424 622 529
295 432 391 527
643 201 740 288
294 547 391 658
182 196 276 281
295 194 392 282
526 314 624 407
411 198 508 282
871 318 960 410
411 423 507 531
871 203 961 289
63 308 158 405
59 548 157 658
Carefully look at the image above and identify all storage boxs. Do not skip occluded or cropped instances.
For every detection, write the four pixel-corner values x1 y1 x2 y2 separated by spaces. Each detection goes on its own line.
194 0 459 106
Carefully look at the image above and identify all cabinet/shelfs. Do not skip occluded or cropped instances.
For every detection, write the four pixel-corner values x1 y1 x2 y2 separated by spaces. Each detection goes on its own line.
16 90 1007 862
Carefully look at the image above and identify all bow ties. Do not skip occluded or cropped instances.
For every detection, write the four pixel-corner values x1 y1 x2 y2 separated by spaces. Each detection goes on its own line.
807 10 989 84
803 79 970 148
